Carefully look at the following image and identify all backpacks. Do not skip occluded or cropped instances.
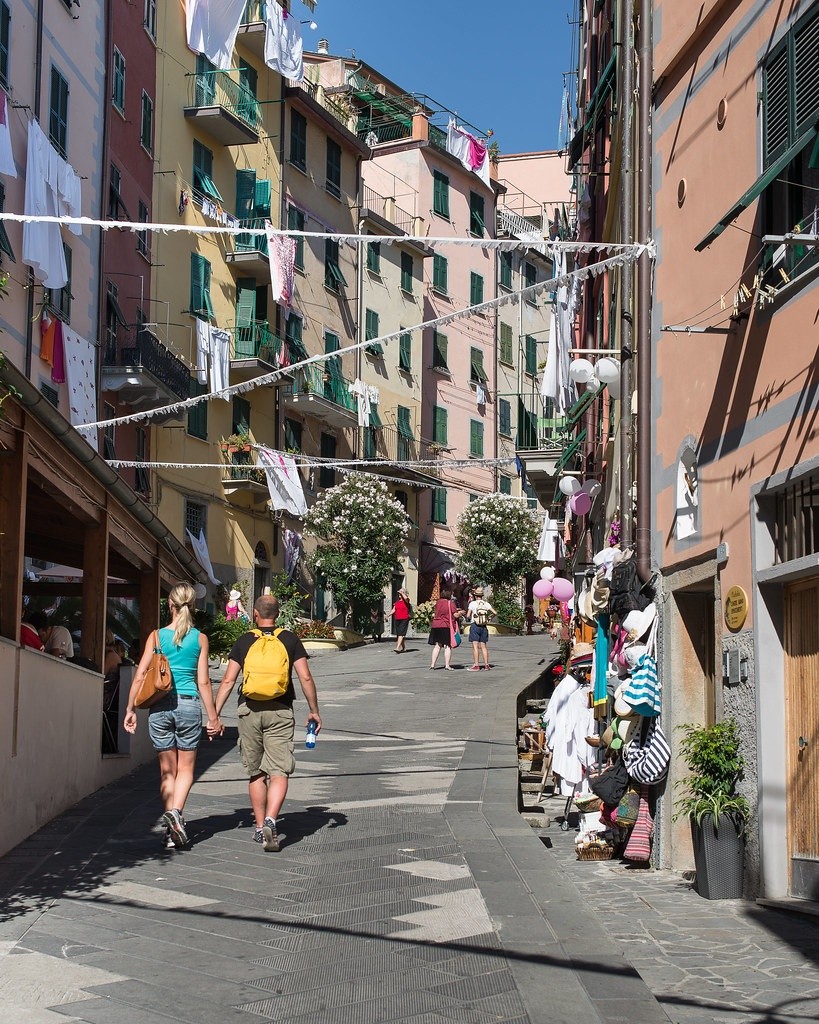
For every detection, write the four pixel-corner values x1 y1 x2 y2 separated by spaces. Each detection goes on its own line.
472 600 489 626
242 627 290 701
409 603 415 620
609 555 651 614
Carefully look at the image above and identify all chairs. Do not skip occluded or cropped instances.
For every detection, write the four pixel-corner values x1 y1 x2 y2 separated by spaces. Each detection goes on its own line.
103 673 121 753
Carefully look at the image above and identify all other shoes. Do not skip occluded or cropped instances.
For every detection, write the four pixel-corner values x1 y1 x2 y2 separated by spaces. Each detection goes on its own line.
445 667 455 672
428 667 435 671
394 649 402 652
402 650 407 652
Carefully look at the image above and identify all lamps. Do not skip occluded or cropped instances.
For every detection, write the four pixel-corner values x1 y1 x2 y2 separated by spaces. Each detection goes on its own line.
299 20 317 30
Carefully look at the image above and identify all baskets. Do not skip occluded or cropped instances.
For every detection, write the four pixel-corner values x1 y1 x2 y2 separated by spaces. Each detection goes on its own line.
574 796 604 812
519 753 546 760
585 737 608 747
576 834 614 861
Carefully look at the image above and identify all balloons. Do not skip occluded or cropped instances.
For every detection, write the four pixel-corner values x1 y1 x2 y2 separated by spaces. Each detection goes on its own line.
532 567 574 602
559 476 602 514
569 357 620 399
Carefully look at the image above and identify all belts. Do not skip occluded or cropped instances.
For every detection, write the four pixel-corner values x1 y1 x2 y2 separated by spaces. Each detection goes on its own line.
180 694 200 699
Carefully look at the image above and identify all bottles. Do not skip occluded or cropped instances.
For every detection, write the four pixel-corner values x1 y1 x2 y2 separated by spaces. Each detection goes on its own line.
305 721 317 748
58 642 66 661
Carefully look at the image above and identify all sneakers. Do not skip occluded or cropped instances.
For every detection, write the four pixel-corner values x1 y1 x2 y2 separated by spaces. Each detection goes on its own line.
161 829 176 848
467 665 481 671
163 809 192 851
485 664 493 670
262 818 280 852
252 826 265 842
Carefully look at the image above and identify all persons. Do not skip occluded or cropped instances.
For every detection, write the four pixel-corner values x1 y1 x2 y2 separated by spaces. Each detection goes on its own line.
20 600 140 713
428 588 466 671
467 587 497 671
384 588 410 652
124 581 321 852
226 590 252 623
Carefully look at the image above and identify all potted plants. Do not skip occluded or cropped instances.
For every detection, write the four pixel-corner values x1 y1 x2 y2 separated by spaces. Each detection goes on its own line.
490 140 501 153
235 459 254 479
220 433 252 453
300 379 314 393
672 717 750 900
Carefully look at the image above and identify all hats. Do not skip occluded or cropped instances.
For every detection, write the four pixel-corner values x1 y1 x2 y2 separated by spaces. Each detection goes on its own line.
621 602 657 653
229 590 241 600
476 587 483 593
578 545 634 627
398 588 409 594
568 642 595 671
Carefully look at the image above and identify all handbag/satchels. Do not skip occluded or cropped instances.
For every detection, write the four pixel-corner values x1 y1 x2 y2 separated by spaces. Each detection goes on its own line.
593 751 654 861
622 616 662 717
134 629 173 709
450 622 462 648
623 713 672 785
